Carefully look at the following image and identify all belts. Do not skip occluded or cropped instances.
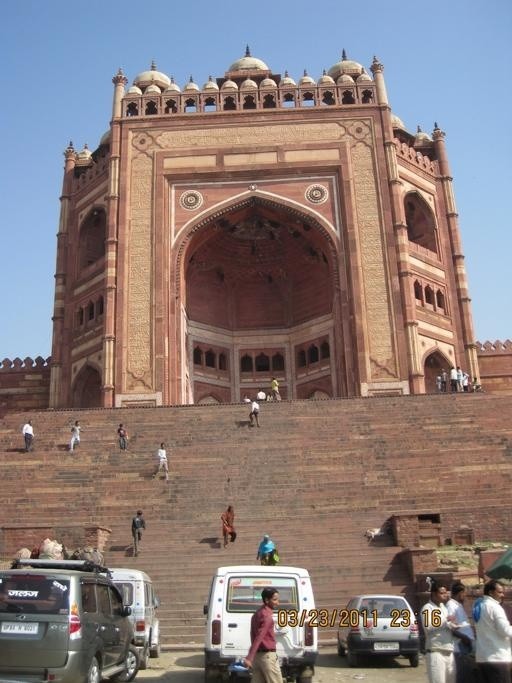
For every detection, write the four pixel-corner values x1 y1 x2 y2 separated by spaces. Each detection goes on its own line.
257 649 276 653
427 648 454 657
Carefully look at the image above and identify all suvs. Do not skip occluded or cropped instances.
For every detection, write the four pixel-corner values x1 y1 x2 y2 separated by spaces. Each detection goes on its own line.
0 559 140 681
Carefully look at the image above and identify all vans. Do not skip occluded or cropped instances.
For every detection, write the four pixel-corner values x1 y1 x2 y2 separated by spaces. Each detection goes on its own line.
336 593 421 668
99 567 161 668
200 563 320 682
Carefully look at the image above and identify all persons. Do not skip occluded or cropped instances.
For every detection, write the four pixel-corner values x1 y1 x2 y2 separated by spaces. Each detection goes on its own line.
69 419 86 452
473 579 512 683
132 509 147 557
23 418 36 452
249 399 261 427
243 586 283 681
433 365 484 393
422 578 461 682
255 533 276 565
241 375 283 402
445 582 474 683
117 423 130 453
220 505 237 549
153 440 169 481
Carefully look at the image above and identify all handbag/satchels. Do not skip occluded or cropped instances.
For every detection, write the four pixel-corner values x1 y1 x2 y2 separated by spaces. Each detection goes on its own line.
458 642 473 655
273 544 280 563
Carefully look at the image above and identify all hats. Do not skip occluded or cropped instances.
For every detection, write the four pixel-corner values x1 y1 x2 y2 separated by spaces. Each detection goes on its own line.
263 534 269 538
